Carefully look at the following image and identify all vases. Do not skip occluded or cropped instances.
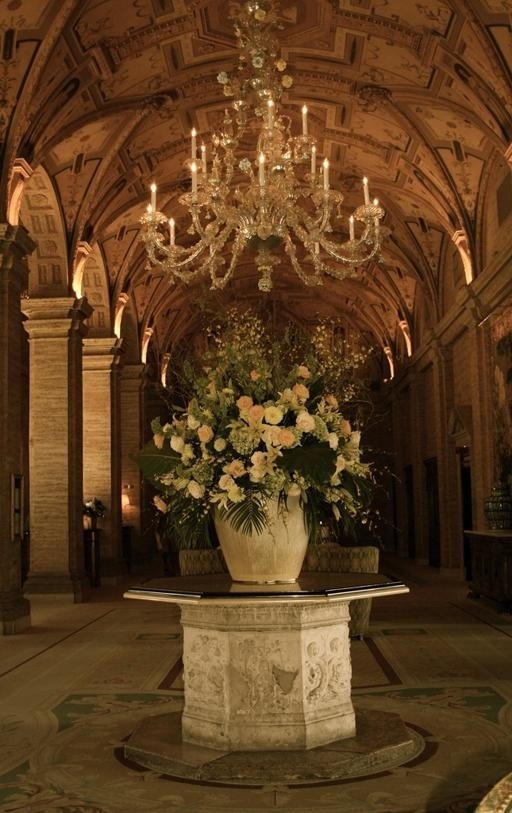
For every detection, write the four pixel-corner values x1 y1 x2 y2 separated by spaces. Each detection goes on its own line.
212 492 311 593
91 517 97 528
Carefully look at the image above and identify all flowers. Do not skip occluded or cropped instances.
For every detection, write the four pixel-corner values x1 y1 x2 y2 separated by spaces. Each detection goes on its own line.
83 495 105 519
127 307 406 551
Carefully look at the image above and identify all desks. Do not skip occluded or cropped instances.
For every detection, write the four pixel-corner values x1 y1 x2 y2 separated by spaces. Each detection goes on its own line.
463 529 512 614
121 571 427 786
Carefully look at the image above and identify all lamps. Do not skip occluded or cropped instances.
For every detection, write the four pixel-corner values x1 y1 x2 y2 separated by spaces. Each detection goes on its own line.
140 0 387 291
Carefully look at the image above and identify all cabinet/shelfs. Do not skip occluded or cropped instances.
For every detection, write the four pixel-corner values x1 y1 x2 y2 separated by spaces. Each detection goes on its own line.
84 528 101 588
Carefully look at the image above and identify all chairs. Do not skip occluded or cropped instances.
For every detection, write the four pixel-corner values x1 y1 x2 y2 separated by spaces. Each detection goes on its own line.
179 549 228 575
303 541 380 640
